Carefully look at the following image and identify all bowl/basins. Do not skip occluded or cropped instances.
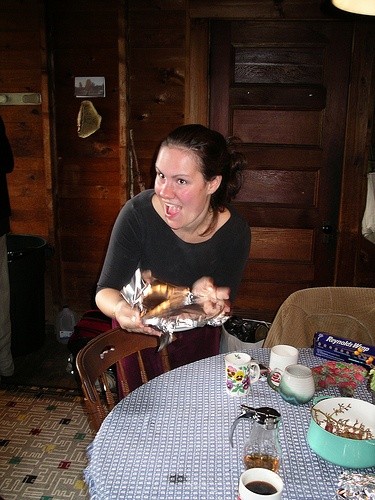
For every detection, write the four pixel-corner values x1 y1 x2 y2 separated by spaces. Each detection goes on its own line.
308 394 375 469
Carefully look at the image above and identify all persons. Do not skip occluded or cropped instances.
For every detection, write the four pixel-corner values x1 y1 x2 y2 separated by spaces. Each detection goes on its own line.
96 124 251 401
0 117 16 385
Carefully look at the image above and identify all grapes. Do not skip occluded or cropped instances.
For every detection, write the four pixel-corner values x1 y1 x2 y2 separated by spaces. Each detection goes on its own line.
325 426 362 440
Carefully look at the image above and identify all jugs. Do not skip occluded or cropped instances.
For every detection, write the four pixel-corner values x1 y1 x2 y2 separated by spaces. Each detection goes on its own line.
228 405 285 473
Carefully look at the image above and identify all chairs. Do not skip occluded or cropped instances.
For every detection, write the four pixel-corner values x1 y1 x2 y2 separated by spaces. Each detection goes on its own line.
75 324 170 433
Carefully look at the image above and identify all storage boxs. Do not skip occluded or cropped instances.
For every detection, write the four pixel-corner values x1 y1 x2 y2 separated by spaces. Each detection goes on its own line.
312 331 375 371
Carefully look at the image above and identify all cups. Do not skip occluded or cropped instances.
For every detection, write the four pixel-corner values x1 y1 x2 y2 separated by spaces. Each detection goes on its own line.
239 468 286 500
267 364 315 405
269 344 298 386
225 352 260 397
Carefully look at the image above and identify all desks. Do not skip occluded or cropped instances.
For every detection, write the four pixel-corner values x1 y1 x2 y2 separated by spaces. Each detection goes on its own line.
87 348 375 499
